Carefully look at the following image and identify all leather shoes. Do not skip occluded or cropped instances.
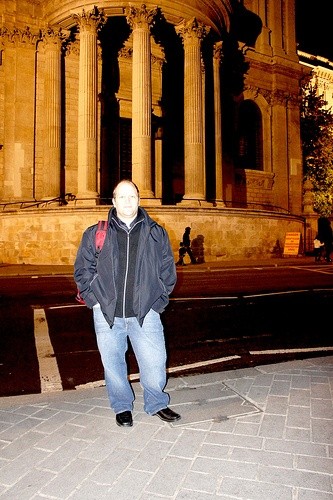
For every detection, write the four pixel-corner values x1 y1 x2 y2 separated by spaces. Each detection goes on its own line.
116 411 133 427
156 408 181 422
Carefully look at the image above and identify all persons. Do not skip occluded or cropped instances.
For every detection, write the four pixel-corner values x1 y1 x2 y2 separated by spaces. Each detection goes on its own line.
313 221 333 263
175 228 197 266
73 180 181 427
